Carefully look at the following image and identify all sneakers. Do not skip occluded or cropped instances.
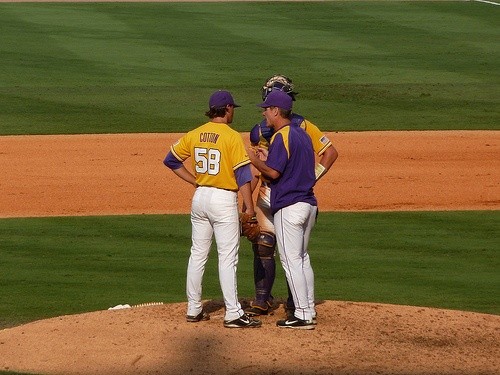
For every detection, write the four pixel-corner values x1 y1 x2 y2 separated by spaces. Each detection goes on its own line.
287 297 296 314
276 315 318 330
186 308 210 322
223 314 262 328
244 294 274 316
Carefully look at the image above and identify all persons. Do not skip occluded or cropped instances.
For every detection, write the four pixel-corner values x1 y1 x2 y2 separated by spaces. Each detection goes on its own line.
240 74 339 316
162 90 261 329
246 90 318 330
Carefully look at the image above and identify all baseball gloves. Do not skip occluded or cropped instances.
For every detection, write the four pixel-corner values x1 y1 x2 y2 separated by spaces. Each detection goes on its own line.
240 211 262 242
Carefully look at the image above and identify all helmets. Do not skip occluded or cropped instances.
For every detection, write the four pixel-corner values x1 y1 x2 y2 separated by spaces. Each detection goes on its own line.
261 74 299 102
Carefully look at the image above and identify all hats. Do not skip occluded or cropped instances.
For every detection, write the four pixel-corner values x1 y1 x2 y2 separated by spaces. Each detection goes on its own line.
209 90 241 111
256 90 293 112
